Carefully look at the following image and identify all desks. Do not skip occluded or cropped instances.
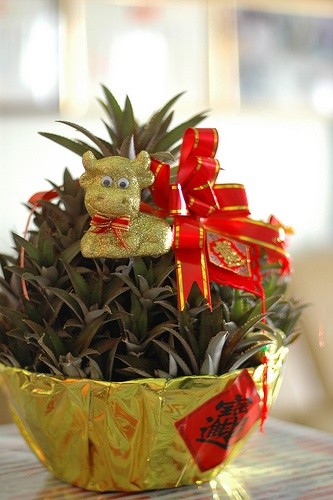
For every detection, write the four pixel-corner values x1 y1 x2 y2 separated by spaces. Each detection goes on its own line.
0 415 333 500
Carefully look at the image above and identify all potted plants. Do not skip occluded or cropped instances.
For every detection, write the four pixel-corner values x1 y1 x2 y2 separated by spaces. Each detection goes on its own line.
0 84 314 496
